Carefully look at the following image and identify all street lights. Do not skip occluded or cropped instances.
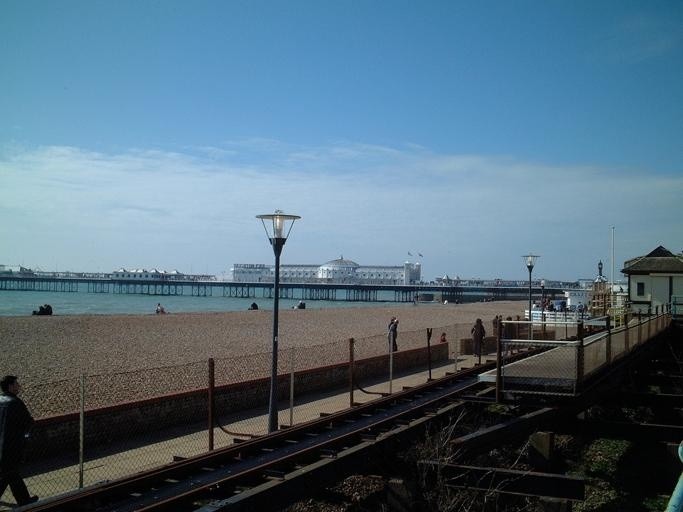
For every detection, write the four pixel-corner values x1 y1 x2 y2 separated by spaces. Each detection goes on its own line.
519 251 543 340
251 206 300 432
541 278 546 311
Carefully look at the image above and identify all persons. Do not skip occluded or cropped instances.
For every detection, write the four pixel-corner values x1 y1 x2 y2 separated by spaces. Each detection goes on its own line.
436 331 448 344
385 315 399 353
489 313 521 336
470 318 486 357
531 298 583 313
0 374 41 507
